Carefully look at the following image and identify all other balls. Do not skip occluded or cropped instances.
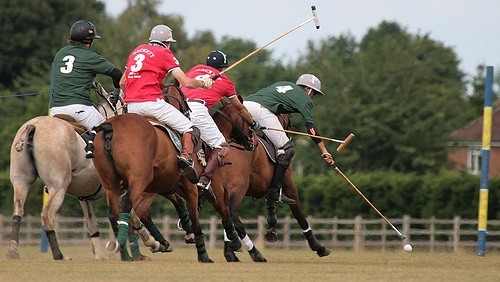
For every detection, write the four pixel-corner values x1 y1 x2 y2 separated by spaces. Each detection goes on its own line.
404 244 412 252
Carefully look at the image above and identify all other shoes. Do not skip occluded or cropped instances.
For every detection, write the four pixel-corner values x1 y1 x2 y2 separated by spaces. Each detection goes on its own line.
177 155 199 184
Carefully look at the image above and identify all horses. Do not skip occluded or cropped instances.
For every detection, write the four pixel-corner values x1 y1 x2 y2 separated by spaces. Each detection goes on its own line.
5 80 165 260
129 94 263 261
84 77 215 264
201 113 332 263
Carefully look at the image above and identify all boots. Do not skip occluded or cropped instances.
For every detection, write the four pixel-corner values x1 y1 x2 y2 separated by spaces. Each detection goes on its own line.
265 165 297 206
196 146 230 202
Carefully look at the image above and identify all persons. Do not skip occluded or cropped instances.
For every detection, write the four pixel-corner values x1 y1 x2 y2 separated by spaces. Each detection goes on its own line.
119 25 213 184
49 21 123 132
180 50 261 204
243 74 335 203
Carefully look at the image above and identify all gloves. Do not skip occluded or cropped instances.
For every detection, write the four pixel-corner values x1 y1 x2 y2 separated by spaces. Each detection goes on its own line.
202 75 216 90
109 90 119 104
250 120 262 132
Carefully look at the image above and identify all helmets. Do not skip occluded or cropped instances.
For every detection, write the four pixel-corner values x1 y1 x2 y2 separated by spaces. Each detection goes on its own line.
69 20 102 42
148 24 177 46
296 73 326 96
206 50 230 68
